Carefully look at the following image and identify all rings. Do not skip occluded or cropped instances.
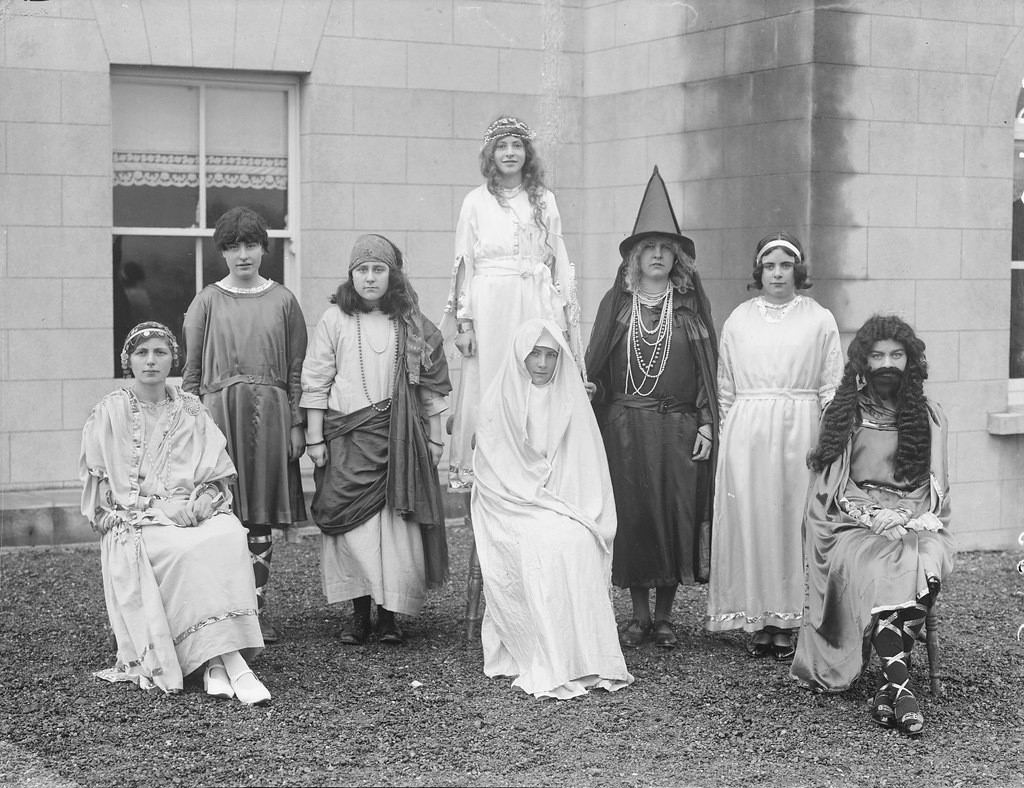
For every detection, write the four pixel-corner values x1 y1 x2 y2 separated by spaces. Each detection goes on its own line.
888 517 893 522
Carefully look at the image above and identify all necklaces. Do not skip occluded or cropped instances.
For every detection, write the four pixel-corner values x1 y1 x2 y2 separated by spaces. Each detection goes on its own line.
624 275 673 396
142 393 173 491
356 308 398 412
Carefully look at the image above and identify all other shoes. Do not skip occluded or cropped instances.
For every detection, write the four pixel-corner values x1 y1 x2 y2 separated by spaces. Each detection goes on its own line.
746 628 773 658
376 620 403 642
620 618 652 646
257 611 278 642
230 670 271 703
870 689 894 728
771 631 795 661
893 695 925 736
340 613 373 644
652 619 678 647
203 664 234 699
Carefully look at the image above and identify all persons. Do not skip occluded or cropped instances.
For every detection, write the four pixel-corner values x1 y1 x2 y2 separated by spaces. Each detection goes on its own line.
712 229 848 659
183 206 309 641
307 235 454 643
476 314 636 701
78 321 272 704
439 116 583 494
797 314 956 736
576 165 720 649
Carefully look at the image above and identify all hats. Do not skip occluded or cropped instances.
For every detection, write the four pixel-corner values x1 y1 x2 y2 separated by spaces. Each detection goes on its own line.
619 164 696 260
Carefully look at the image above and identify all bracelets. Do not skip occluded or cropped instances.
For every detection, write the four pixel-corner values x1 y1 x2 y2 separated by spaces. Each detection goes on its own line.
429 439 444 446
701 427 713 438
697 431 712 441
305 439 325 446
